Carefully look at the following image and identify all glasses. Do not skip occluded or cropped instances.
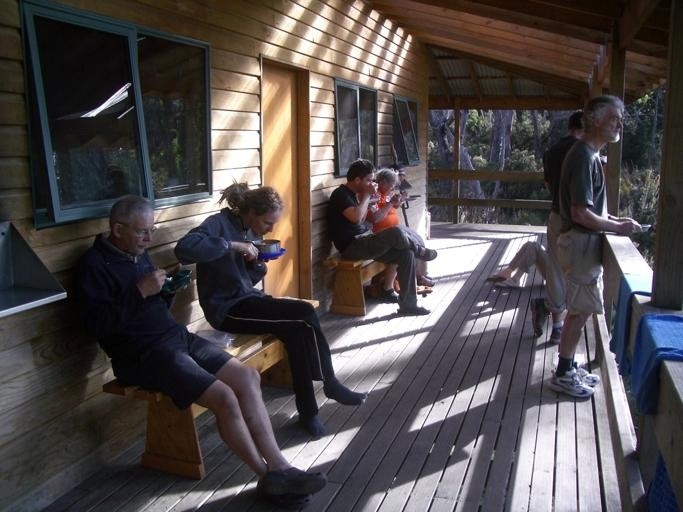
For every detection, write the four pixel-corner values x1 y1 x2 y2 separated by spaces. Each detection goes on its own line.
608 114 627 125
115 220 158 237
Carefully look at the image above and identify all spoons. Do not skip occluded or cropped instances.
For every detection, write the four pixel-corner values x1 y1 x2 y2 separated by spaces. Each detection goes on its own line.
162 276 173 281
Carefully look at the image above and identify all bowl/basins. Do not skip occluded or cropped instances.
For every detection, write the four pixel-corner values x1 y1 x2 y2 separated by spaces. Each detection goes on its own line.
251 238 281 254
162 270 191 292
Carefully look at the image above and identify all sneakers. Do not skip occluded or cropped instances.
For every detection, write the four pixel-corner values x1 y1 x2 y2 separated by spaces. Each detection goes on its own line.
394 280 401 292
548 370 596 399
257 474 310 510
424 274 433 280
531 297 549 337
322 386 368 405
300 416 326 437
416 276 435 287
284 466 329 502
550 327 563 344
575 364 600 388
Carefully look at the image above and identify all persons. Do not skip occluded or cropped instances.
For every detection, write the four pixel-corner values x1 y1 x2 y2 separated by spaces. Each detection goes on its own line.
359 168 436 287
327 159 437 315
486 240 546 289
530 110 585 344
547 94 642 398
67 196 326 506
174 183 367 436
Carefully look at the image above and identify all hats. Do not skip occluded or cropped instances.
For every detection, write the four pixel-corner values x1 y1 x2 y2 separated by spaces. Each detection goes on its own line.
398 169 406 176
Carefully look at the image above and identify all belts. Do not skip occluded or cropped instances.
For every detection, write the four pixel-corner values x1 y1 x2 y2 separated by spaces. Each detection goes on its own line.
339 240 352 253
551 207 561 216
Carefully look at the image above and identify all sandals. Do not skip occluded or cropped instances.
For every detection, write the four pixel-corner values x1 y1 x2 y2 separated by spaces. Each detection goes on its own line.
380 286 400 302
413 244 437 262
495 278 521 288
399 304 430 317
486 270 510 281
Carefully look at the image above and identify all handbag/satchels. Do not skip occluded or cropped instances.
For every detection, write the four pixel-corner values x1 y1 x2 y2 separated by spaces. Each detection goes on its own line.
370 273 401 299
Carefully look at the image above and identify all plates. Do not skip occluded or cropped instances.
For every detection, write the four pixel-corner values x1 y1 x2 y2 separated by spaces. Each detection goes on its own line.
633 225 650 233
368 197 380 204
254 249 285 260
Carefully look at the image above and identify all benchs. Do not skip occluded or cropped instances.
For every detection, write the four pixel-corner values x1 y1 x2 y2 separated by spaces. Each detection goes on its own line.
322 252 385 316
101 295 320 479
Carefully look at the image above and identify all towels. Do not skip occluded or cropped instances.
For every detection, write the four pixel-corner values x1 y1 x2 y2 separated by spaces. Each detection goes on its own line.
610 276 650 375
631 314 683 413
